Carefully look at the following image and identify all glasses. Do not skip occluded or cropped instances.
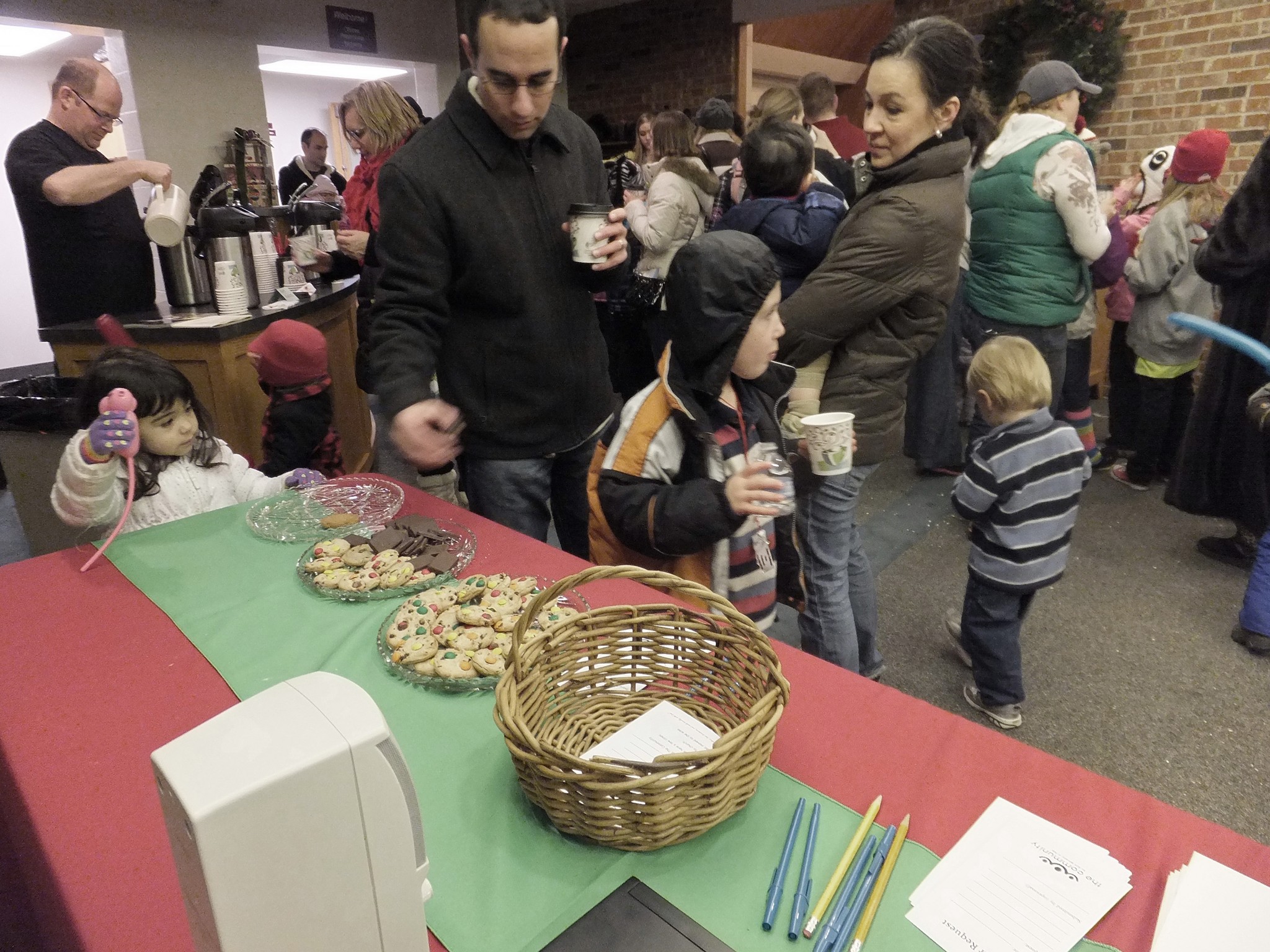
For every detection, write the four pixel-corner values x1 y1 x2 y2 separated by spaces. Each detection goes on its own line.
347 124 368 145
475 59 563 96
72 89 124 126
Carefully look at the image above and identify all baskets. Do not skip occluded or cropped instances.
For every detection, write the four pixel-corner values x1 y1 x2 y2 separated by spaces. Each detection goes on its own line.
493 564 789 853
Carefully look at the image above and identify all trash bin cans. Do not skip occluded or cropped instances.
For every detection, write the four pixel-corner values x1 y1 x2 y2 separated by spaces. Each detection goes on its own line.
0 376 114 559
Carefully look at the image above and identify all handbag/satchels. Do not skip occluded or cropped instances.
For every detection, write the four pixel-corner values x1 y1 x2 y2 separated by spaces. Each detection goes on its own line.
605 157 641 207
626 275 667 318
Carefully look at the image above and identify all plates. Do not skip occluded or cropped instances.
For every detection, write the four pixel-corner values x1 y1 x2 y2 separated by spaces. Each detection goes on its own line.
246 477 405 545
377 574 592 691
296 517 477 601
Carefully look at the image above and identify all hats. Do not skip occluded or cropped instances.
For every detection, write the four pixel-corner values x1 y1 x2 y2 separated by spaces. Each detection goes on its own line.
248 319 331 399
1016 61 1103 111
1172 130 1230 184
1137 146 1177 210
1075 116 1096 141
697 97 735 129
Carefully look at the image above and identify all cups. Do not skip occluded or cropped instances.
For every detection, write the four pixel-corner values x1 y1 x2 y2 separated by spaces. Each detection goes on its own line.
800 412 856 476
213 261 250 316
566 202 614 264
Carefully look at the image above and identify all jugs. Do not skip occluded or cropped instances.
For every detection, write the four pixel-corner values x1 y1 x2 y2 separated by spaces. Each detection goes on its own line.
193 182 261 309
291 201 342 285
158 225 212 306
144 184 191 248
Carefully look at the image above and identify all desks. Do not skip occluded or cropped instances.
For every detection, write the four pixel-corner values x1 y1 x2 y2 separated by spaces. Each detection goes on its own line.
0 462 1270 952
37 271 379 480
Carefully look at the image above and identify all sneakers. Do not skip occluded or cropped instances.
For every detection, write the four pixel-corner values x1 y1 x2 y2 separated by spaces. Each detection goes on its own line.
1197 529 1256 569
963 684 1022 729
1111 464 1149 491
1232 624 1270 655
944 620 973 667
864 664 886 680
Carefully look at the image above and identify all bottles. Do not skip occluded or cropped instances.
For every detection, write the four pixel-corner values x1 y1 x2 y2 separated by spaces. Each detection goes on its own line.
752 441 796 516
249 229 338 307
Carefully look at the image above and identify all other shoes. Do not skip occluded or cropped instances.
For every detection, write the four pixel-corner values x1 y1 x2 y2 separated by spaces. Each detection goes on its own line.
931 464 963 475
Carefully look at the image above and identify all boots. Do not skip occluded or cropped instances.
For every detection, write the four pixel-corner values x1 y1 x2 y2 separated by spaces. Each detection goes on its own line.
1065 407 1103 466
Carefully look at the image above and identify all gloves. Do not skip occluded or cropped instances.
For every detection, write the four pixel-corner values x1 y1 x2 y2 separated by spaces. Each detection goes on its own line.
79 410 135 463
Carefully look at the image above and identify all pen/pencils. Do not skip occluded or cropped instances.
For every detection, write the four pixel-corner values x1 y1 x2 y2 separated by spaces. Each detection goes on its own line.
761 797 806 931
802 795 883 940
814 835 875 952
788 803 820 941
832 823 895 952
849 813 911 952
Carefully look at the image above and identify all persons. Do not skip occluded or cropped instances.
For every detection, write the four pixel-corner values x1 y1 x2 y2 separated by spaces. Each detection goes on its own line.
246 318 347 479
1232 383 1270 649
279 128 347 205
51 346 327 539
367 0 637 560
747 85 856 211
4 59 173 328
798 71 870 160
1109 129 1230 491
633 113 657 163
773 16 999 682
951 335 1092 729
588 231 857 632
622 110 720 398
713 121 846 301
292 78 469 510
1164 137 1270 569
1098 146 1176 470
964 60 1128 465
695 98 740 233
307 174 348 229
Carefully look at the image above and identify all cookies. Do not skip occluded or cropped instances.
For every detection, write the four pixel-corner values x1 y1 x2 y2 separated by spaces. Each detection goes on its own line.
343 513 459 576
385 571 577 679
303 538 438 593
321 513 359 528
